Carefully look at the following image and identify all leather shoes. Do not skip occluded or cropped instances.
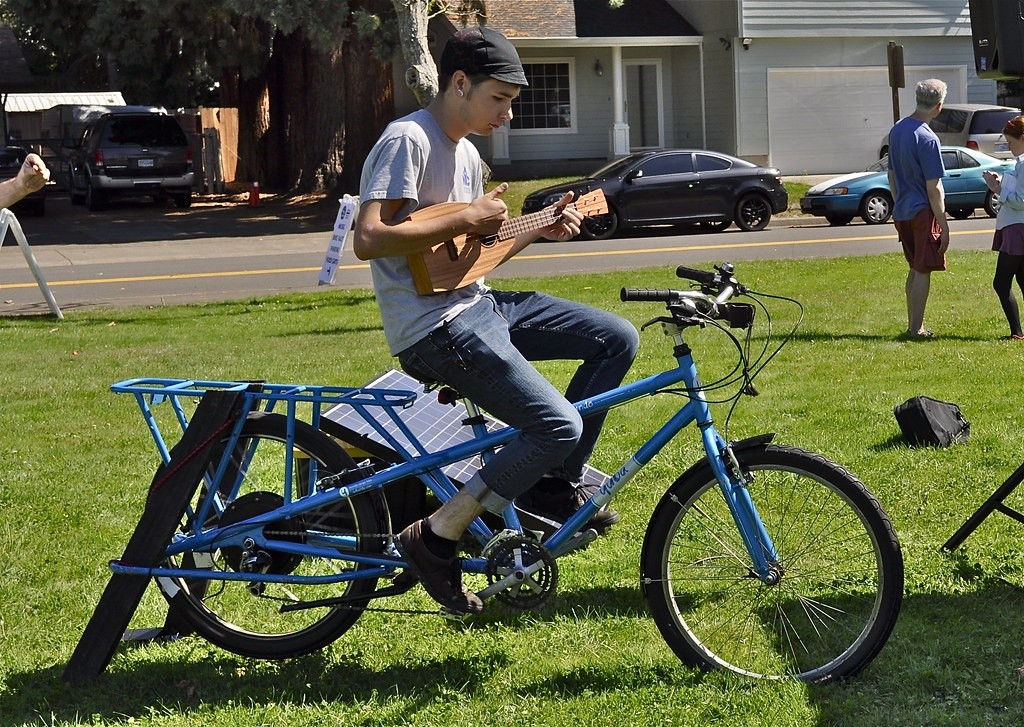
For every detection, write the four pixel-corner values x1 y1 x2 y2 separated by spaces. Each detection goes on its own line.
392 519 485 613
519 482 618 527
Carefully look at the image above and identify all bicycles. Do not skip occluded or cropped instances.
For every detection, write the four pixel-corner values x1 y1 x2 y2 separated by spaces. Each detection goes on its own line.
115 258 905 694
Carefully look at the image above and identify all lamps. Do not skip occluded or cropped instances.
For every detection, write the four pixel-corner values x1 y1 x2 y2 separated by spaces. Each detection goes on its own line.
595 59 603 76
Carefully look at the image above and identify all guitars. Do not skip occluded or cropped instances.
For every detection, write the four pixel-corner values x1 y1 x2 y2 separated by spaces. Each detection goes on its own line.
397 184 609 297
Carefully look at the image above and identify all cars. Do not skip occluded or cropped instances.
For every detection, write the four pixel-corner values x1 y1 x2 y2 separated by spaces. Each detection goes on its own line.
66 105 192 207
516 146 789 234
799 144 1014 226
870 103 1023 161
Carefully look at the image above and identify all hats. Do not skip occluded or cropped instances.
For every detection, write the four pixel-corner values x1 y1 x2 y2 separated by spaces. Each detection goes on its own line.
441 26 529 86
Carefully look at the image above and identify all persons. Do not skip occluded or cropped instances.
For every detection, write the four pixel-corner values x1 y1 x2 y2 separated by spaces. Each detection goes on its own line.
983 114 1024 340
353 27 641 612
0 152 51 211
885 78 950 340
